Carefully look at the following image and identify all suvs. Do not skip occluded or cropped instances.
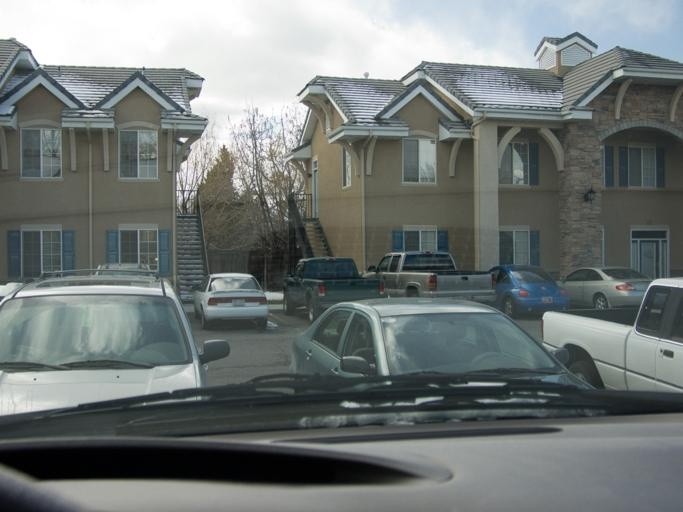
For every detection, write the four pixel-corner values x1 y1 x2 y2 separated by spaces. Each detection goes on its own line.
94 262 154 277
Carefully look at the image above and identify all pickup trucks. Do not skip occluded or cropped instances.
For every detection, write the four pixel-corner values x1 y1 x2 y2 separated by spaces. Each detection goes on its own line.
281 257 385 325
360 252 498 305
541 276 683 392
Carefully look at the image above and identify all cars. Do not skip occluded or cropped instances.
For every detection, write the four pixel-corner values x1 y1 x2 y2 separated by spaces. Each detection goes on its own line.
192 272 269 330
483 265 569 319
555 266 668 310
1 268 232 416
291 296 599 393
